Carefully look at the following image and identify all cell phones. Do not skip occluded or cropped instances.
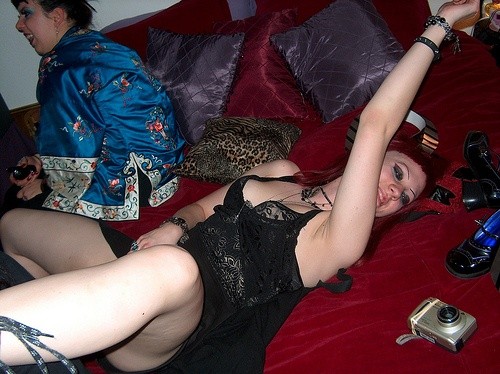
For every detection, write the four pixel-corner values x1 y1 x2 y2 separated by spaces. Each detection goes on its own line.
6 165 35 179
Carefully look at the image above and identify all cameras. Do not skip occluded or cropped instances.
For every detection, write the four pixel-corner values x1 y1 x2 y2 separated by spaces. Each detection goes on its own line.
407 296 478 353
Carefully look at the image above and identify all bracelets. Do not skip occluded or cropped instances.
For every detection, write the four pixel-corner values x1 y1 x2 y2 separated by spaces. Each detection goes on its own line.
424 16 462 55
161 216 191 243
413 37 441 62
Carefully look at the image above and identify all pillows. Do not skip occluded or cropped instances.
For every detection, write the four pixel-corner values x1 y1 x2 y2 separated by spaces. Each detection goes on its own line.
143 0 407 185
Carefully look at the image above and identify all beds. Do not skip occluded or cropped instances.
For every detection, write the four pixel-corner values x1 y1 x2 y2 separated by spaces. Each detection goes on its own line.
93 0 500 374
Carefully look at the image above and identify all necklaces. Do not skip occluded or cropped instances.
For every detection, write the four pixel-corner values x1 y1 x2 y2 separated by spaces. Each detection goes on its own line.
300 184 333 211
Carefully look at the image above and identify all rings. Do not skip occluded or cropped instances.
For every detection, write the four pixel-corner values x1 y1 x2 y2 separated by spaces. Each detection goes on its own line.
132 245 138 252
131 240 137 245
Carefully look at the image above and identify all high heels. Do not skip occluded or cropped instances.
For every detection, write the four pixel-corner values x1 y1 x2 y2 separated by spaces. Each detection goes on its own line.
446 213 500 278
457 132 500 212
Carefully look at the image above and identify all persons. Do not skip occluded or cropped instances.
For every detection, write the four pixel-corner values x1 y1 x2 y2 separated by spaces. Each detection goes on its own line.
0 0 479 374
0 0 184 221
